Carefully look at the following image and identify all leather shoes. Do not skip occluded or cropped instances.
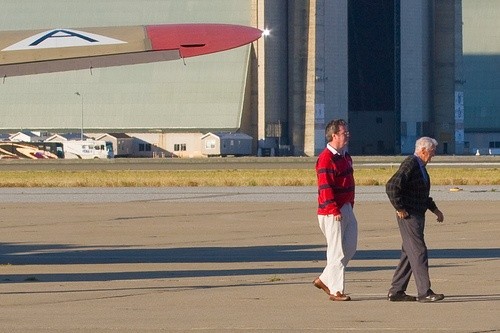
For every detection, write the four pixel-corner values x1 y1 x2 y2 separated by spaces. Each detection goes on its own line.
329 292 350 302
313 277 331 295
388 292 416 302
417 289 444 303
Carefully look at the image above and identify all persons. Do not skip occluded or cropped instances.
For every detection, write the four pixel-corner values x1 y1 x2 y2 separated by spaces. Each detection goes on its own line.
385 136 445 303
313 118 357 302
